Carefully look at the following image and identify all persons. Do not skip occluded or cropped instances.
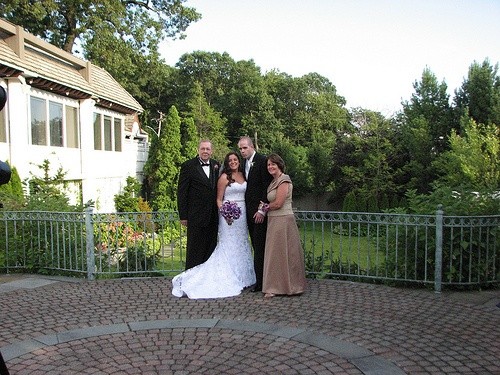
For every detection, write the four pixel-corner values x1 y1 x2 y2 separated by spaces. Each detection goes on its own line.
258 153 308 298
178 140 225 271
237 137 274 292
171 152 256 299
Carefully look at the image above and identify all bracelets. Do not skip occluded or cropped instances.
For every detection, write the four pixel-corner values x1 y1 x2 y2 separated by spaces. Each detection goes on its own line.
261 203 270 212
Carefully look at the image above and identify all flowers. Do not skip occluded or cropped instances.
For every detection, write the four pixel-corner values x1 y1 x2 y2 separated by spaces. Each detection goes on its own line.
258 201 269 212
220 200 241 226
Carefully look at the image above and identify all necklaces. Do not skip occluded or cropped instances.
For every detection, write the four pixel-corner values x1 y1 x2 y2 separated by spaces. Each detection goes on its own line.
272 173 283 183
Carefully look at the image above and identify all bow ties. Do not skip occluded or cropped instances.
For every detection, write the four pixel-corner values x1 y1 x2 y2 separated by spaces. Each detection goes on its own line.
199 158 210 166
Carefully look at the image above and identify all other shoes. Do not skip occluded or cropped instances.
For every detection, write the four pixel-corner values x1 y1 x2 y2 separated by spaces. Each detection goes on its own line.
264 293 275 297
250 284 262 292
